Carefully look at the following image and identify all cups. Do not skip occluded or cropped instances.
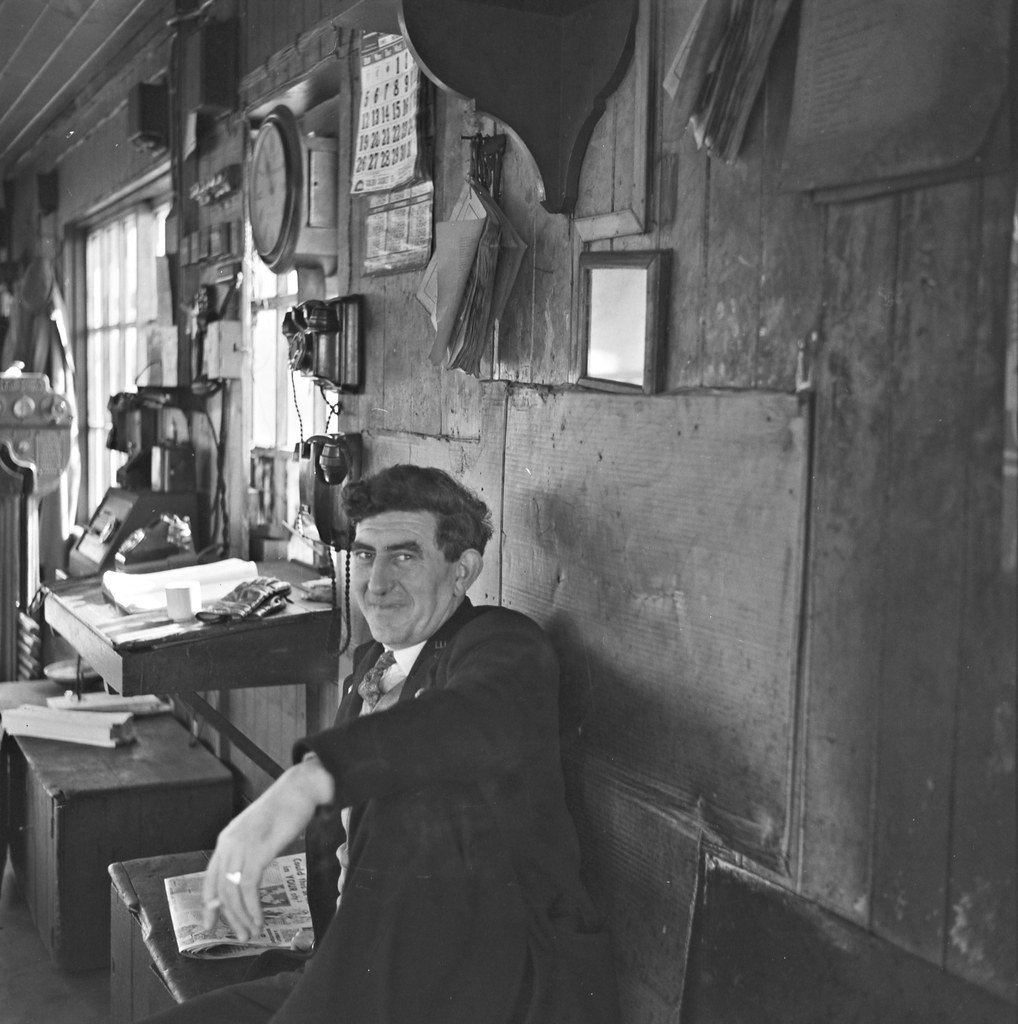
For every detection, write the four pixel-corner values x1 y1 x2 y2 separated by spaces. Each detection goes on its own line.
165 581 200 623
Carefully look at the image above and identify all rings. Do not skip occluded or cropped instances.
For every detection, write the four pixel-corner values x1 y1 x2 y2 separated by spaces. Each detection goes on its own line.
224 871 243 885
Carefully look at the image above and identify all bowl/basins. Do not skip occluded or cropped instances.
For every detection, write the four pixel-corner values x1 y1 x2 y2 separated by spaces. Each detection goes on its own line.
44 660 99 689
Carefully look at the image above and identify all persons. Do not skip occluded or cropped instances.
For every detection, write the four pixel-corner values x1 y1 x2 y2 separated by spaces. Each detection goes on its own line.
130 462 622 1024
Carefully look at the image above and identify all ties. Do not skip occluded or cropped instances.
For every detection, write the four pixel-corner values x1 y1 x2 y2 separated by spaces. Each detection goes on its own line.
358 651 397 709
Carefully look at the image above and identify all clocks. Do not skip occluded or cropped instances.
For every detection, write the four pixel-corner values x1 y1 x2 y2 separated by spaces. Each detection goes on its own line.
247 104 302 275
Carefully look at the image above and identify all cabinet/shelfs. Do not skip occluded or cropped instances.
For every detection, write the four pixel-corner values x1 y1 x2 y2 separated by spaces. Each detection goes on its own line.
0 707 235 973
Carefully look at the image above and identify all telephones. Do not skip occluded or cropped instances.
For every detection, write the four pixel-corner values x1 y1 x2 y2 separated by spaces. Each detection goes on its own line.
282 289 365 394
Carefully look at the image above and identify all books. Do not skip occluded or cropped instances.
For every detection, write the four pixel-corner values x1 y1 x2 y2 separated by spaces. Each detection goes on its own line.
414 175 530 378
662 0 795 165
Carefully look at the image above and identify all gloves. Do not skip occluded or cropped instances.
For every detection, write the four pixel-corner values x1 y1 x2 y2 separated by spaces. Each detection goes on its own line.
194 576 292 625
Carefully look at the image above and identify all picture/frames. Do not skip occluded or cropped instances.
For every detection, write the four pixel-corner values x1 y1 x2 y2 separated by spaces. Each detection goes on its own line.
573 250 672 397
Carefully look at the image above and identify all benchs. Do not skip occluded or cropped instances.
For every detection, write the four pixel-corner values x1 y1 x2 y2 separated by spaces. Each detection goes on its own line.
108 839 313 1023
